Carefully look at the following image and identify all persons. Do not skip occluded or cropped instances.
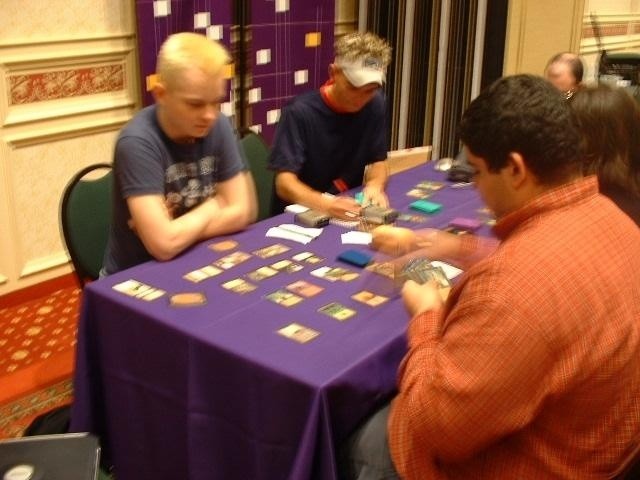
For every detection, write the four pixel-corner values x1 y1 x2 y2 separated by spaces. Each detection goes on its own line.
267 30 390 220
98 32 260 279
543 51 584 96
348 73 640 480
572 84 640 225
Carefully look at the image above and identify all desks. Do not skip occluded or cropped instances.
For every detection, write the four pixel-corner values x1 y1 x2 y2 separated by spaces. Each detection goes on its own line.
81 160 496 480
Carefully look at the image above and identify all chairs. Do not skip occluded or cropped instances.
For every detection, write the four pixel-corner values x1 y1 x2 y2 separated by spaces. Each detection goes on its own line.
61 129 278 280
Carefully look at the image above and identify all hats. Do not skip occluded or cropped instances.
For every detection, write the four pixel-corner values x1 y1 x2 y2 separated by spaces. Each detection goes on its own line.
332 33 393 89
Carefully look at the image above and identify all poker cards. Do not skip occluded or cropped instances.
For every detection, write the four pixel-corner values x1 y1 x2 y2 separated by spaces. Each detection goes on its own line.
112 182 500 345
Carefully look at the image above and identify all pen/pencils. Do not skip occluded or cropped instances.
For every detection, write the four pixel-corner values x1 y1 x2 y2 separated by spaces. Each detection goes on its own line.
334 179 353 199
